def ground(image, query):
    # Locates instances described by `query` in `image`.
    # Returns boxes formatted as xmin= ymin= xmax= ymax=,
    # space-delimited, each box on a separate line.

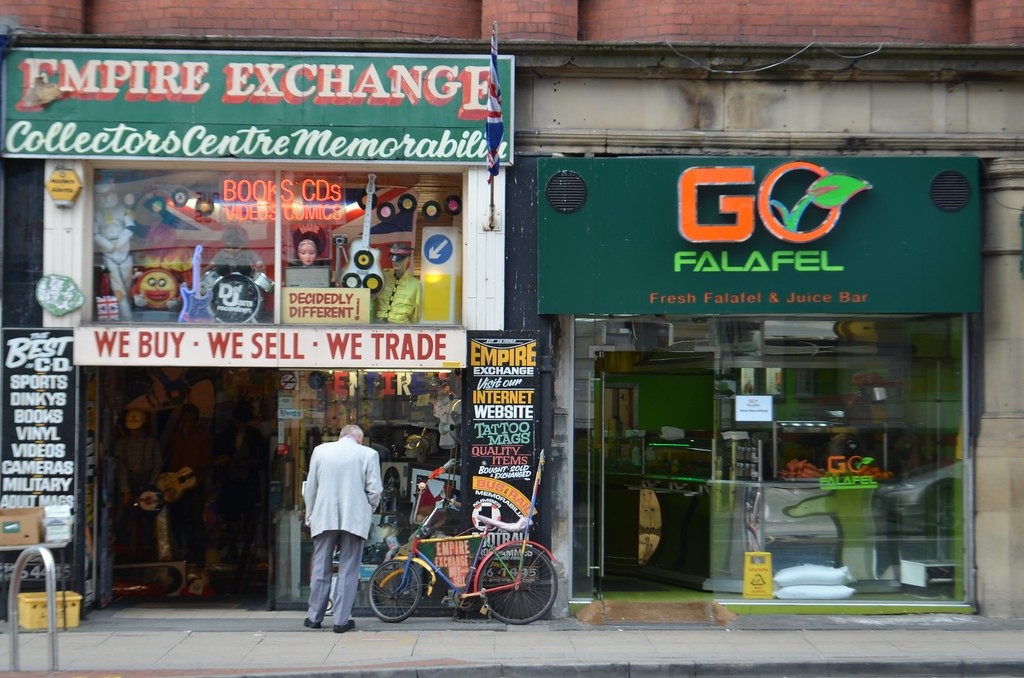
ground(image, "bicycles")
xmin=367 ymin=481 xmax=559 ymax=625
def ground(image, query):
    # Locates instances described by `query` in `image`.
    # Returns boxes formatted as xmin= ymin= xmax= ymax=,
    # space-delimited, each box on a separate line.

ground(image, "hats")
xmin=125 ymin=411 xmax=146 ymax=429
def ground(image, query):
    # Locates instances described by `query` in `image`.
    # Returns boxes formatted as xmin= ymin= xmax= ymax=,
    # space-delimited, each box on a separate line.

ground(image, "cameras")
xmin=333 ymin=235 xmax=348 ymax=245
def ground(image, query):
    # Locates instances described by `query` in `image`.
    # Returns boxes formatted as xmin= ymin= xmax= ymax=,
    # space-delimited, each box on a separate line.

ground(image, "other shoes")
xmin=221 ymin=547 xmax=253 ymax=564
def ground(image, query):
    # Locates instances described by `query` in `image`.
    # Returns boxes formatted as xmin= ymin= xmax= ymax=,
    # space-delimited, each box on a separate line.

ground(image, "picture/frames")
xmin=379 ymin=457 xmax=462 ymax=505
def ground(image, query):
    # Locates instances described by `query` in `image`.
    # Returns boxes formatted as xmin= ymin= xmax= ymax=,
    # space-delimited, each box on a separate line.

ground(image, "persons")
xmin=201 ymin=228 xmax=266 ymax=278
xmin=93 ymin=182 xmax=134 ymax=319
xmin=298 ymin=239 xmax=422 ymax=325
xmin=113 ymin=404 xmax=270 ymax=578
xmin=303 ymin=425 xmax=384 ymax=633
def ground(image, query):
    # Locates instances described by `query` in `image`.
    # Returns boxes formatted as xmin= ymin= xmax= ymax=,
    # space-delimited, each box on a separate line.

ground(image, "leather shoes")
xmin=333 ymin=620 xmax=354 ymax=633
xmin=305 ymin=618 xmax=321 ymax=629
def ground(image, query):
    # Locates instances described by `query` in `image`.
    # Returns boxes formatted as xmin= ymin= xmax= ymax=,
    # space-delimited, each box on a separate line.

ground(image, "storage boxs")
xmin=19 ymin=590 xmax=83 ymax=629
xmin=0 ymin=507 xmax=43 ymax=545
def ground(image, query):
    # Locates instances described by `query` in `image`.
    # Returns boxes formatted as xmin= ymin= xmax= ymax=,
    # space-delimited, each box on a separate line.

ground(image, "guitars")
xmin=338 ymin=173 xmax=386 ymax=296
xmin=177 ymin=244 xmax=215 ymax=323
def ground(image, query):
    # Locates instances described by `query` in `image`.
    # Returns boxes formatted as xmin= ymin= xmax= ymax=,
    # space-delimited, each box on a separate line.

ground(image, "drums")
xmin=252 ymin=273 xmax=275 ymax=295
xmin=199 ymin=270 xmax=220 ymax=293
xmin=212 ymin=272 xmax=261 ymax=324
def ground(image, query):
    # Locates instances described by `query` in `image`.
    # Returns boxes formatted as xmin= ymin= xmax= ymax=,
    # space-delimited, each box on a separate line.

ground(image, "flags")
xmin=486 ymin=21 xmax=503 ymax=185
